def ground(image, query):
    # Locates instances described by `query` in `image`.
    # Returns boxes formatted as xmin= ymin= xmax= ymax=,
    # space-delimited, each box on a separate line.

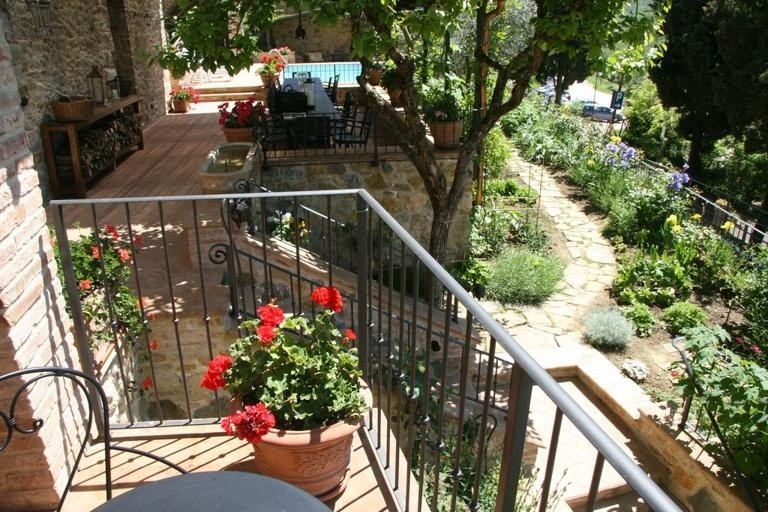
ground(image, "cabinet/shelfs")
xmin=40 ymin=94 xmax=143 ymax=200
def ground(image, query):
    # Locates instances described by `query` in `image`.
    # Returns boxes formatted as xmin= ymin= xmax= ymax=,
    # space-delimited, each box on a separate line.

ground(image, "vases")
xmin=173 ymin=100 xmax=188 ymax=113
xmin=251 ymin=378 xmax=373 ymax=504
xmin=282 ymin=55 xmax=288 ymax=62
xmin=260 ymin=75 xmax=278 ymax=87
xmin=222 ymin=127 xmax=254 ymax=143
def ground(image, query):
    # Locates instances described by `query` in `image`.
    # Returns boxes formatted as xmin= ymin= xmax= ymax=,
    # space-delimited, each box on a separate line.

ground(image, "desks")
xmin=282 ymin=61 xmax=363 ymax=85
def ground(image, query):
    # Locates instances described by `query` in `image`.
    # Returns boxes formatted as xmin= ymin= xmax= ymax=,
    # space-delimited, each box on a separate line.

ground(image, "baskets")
xmin=49 ymin=96 xmax=96 ymax=123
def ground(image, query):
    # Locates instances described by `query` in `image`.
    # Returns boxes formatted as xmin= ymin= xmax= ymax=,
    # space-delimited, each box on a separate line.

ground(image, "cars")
xmin=537 ymin=84 xmax=624 ymax=124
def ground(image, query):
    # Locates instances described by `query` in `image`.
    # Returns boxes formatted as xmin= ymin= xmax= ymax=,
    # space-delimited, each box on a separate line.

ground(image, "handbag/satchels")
xmin=273 ymin=91 xmax=308 ymax=112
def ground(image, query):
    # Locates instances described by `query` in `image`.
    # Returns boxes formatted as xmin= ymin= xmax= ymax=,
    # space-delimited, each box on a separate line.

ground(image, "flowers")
xmin=202 ymin=286 xmax=376 ymax=444
xmin=170 ymin=84 xmax=200 ymax=105
xmin=218 ymin=98 xmax=267 ymax=127
xmin=254 ymin=54 xmax=286 ymax=75
xmin=279 ymin=46 xmax=289 ymax=55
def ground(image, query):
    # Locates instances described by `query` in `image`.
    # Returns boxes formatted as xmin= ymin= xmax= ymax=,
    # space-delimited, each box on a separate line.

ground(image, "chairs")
xmin=0 ymin=366 xmax=334 ymax=512
xmin=259 ymin=72 xmax=376 ymax=152
xmin=305 ymin=51 xmax=345 ymax=62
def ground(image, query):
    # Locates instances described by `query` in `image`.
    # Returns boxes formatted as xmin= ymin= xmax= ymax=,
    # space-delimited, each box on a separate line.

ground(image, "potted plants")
xmin=367 ymin=62 xmax=465 ymax=149
